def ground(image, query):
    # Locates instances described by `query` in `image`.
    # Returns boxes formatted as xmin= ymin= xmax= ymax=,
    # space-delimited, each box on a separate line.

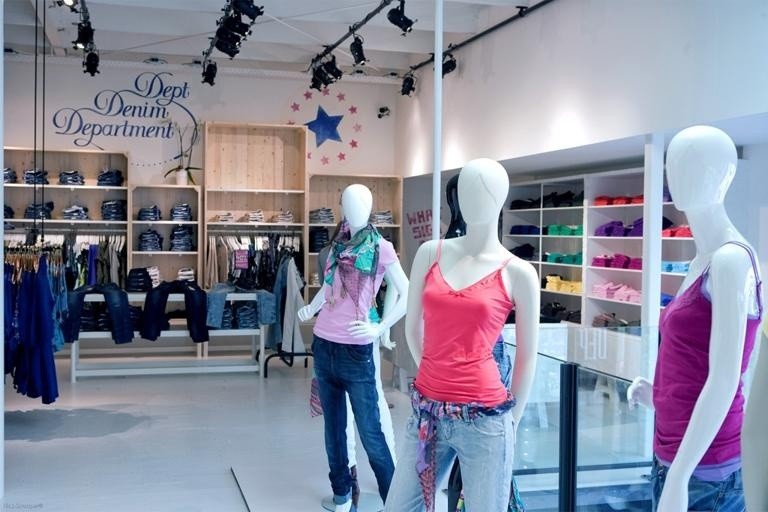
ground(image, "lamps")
xmin=56 ymin=0 xmax=100 ymax=77
xmin=309 ymin=0 xmax=417 ymax=96
xmin=442 ymin=51 xmax=456 ymax=79
xmin=201 ymin=0 xmax=264 ymax=86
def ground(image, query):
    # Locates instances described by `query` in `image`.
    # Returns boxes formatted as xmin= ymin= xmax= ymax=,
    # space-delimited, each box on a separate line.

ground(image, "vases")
xmin=173 ymin=144 xmax=192 ymax=185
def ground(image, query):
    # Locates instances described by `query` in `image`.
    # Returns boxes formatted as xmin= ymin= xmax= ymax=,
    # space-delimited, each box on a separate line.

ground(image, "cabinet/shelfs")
xmin=307 ymin=171 xmax=404 ymax=305
xmin=129 ymin=185 xmax=202 ymax=288
xmin=70 ymin=291 xmax=270 ymax=384
xmin=203 ymin=120 xmax=309 ymax=303
xmin=2 ymin=145 xmax=131 ymax=279
xmin=499 ymin=165 xmax=696 ymax=382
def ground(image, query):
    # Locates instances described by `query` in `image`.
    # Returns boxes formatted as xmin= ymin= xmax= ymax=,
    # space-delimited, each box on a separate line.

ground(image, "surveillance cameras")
xmin=378 ymin=106 xmax=390 ymax=118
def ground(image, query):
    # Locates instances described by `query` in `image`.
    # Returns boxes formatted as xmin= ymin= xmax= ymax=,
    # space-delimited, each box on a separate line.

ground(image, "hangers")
xmin=3 ymin=228 xmax=126 ymax=285
xmin=208 ymin=229 xmax=301 ymax=253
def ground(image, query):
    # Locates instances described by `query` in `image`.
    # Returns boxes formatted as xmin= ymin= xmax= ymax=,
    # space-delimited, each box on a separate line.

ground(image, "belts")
xmin=410 ymin=380 xmax=517 ymax=512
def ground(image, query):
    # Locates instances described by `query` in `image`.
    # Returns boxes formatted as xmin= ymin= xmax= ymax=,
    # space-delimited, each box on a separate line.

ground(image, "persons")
xmin=625 ymin=124 xmax=763 ymax=511
xmin=380 ymin=155 xmax=539 ymax=511
xmin=293 ymin=181 xmax=410 ymax=511
